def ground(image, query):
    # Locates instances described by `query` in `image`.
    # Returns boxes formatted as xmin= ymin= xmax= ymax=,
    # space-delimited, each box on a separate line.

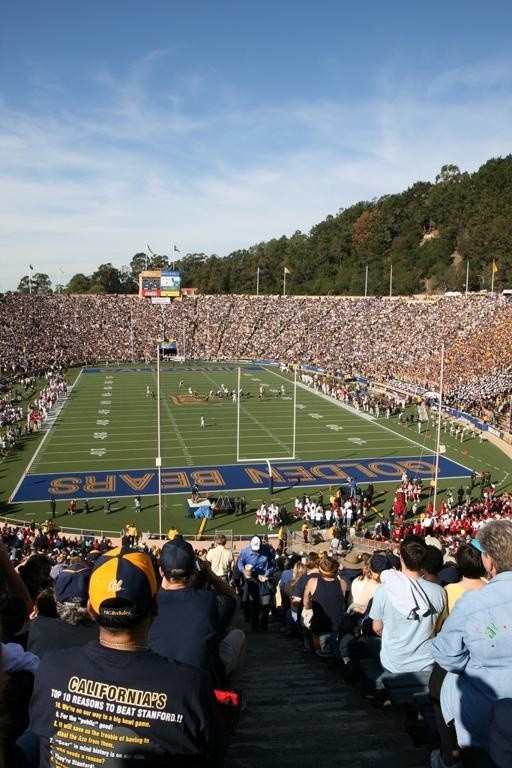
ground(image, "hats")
xmin=88 ymin=545 xmax=158 ymax=616
xmin=339 ymin=551 xmax=364 ymax=570
xmin=159 ymin=538 xmax=195 ymax=578
xmin=471 ymin=538 xmax=486 ymax=553
xmin=54 ymin=561 xmax=92 ymax=607
xmin=250 ymin=534 xmax=262 ymax=551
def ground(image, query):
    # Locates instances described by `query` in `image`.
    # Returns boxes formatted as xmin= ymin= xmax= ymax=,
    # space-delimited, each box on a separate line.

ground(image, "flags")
xmin=492 ymin=258 xmax=500 ymax=273
xmin=28 ymin=263 xmax=34 ymax=273
xmin=57 ymin=263 xmax=65 ymax=275
xmin=284 ymin=267 xmax=292 ymax=275
xmin=173 ymin=241 xmax=181 ymax=255
xmin=145 ymin=242 xmax=152 ymax=255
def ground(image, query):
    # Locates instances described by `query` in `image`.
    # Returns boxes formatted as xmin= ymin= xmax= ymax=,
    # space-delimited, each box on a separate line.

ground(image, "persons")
xmin=28 ymin=590 xmax=60 ymax=622
xmin=421 ymin=545 xmax=444 ymax=587
xmin=18 ymin=564 xmax=91 ymax=659
xmin=368 ymin=534 xmax=450 ymax=694
xmin=431 ymin=520 xmax=512 ymax=767
xmin=147 ymin=540 xmax=246 ymax=693
xmin=0 ymin=517 xmax=207 ymax=569
xmin=444 ymin=542 xmax=488 ymax=616
xmin=305 ymin=552 xmax=321 ymax=575
xmin=273 ymin=557 xmax=291 ymax=594
xmin=206 ymin=535 xmax=235 ymax=586
xmin=279 ymin=555 xmax=302 ymax=603
xmin=302 ymin=556 xmax=347 ymax=658
xmin=340 ymin=553 xmax=365 ymax=575
xmin=279 ymin=561 xmax=307 ymax=640
xmin=28 ymin=545 xmax=230 ymax=768
xmin=44 ymin=484 xmax=248 ymax=518
xmin=337 ymin=554 xmax=392 ymax=641
xmin=0 ymin=536 xmax=39 ymax=621
xmin=254 ymin=469 xmax=512 ymax=554
xmin=238 ymin=536 xmax=279 ymax=631
xmin=0 ymin=289 xmax=512 ymax=458
